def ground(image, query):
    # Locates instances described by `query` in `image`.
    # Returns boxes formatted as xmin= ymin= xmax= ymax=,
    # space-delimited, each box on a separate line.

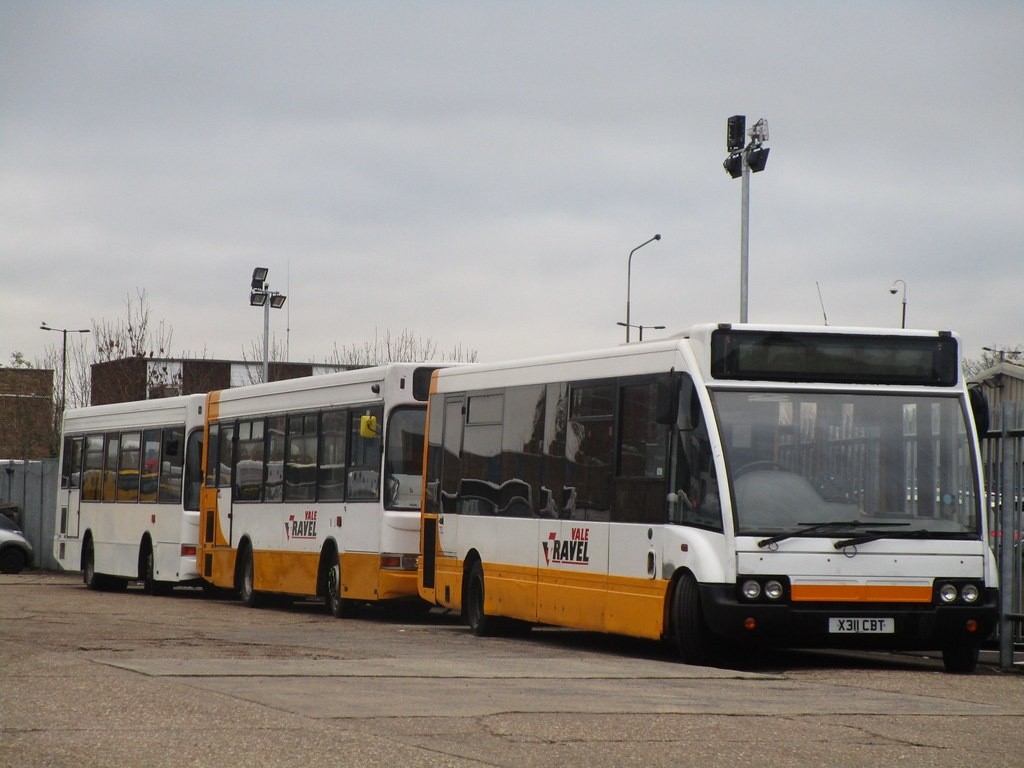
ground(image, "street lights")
xmin=249 ymin=266 xmax=287 ymax=383
xmin=627 ymin=233 xmax=662 ymax=343
xmin=890 ymin=278 xmax=907 ymax=329
xmin=40 ymin=326 xmax=91 ymax=415
xmin=616 ymin=322 xmax=665 ymax=342
xmin=981 ymin=347 xmax=1021 ymax=363
xmin=6 ymin=468 xmax=15 ymax=504
xmin=723 ymin=116 xmax=770 ymax=321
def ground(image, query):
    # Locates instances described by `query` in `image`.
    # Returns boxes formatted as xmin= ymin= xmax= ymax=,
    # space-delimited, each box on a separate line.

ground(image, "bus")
xmin=415 ymin=321 xmax=1000 ymax=674
xmin=196 ymin=363 xmax=474 ymax=617
xmin=53 ymin=394 xmax=210 ymax=595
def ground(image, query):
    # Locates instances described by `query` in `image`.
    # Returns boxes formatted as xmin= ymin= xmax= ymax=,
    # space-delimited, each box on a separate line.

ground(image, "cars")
xmin=0 ymin=513 xmax=35 ymax=573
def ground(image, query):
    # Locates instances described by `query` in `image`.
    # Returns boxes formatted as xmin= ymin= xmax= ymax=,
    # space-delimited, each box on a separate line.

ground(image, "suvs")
xmin=849 ymin=484 xmax=1024 ymax=548
